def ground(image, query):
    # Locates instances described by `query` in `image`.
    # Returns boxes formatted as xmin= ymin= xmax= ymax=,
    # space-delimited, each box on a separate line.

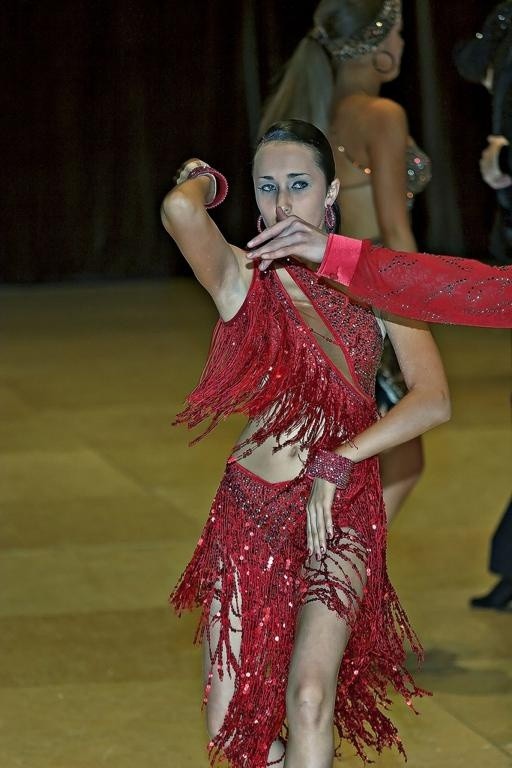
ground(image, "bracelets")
xmin=197 ymin=172 xmax=218 ymax=206
xmin=304 ymin=447 xmax=355 ymax=493
xmin=183 ymin=165 xmax=230 ymax=211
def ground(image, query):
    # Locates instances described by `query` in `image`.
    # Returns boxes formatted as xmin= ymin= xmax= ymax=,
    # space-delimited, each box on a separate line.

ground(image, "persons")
xmin=254 ymin=0 xmax=443 ymax=535
xmin=468 ymin=500 xmax=512 ymax=613
xmin=478 ymin=133 xmax=512 ymax=192
xmin=242 ymin=205 xmax=512 ymax=334
xmin=155 ymin=116 xmax=458 ymax=768
